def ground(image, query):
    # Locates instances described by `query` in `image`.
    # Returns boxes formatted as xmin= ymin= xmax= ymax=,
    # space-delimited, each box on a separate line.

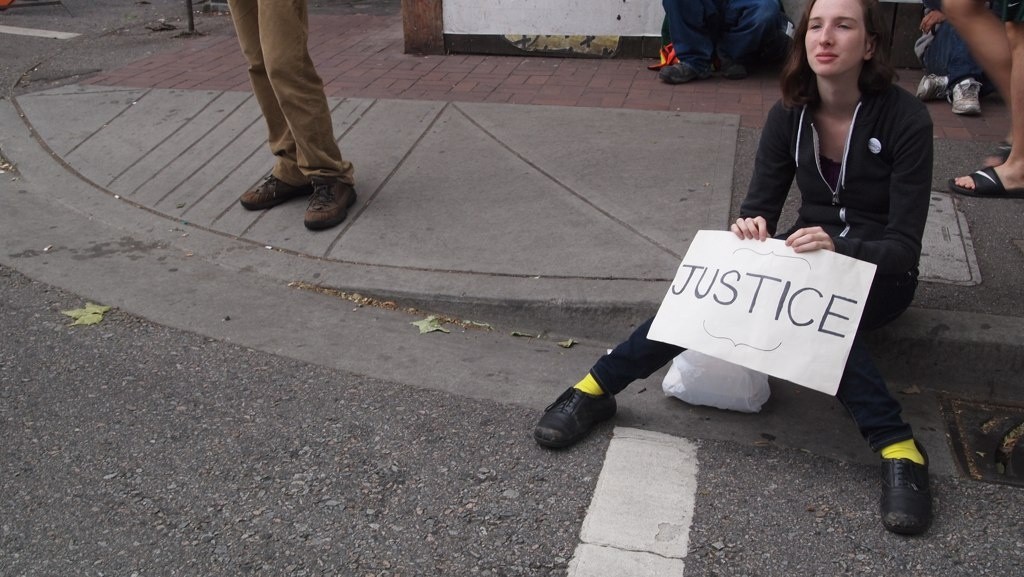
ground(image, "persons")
xmin=225 ymin=0 xmax=356 ymax=230
xmin=534 ymin=0 xmax=933 ymax=536
xmin=941 ymin=0 xmax=1024 ymax=199
xmin=659 ymin=0 xmax=808 ymax=84
xmin=915 ymin=0 xmax=1003 ymax=114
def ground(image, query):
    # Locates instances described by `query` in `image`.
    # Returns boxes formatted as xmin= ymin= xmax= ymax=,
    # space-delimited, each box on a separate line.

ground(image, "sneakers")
xmin=304 ymin=180 xmax=358 ymax=230
xmin=952 ymin=77 xmax=983 ymax=116
xmin=240 ymin=174 xmax=314 ymax=211
xmin=659 ymin=61 xmax=713 ymax=85
xmin=915 ymin=73 xmax=952 ymax=104
xmin=720 ymin=64 xmax=748 ymax=80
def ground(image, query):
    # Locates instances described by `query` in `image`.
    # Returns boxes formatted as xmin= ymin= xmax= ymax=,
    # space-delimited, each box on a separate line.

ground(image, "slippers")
xmin=986 ymin=138 xmax=1013 ymax=165
xmin=948 ymin=164 xmax=1024 ymax=199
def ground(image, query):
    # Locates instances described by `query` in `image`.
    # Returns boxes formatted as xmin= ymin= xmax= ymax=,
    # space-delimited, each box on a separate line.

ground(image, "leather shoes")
xmin=534 ymin=386 xmax=616 ymax=449
xmin=880 ymin=440 xmax=934 ymax=535
